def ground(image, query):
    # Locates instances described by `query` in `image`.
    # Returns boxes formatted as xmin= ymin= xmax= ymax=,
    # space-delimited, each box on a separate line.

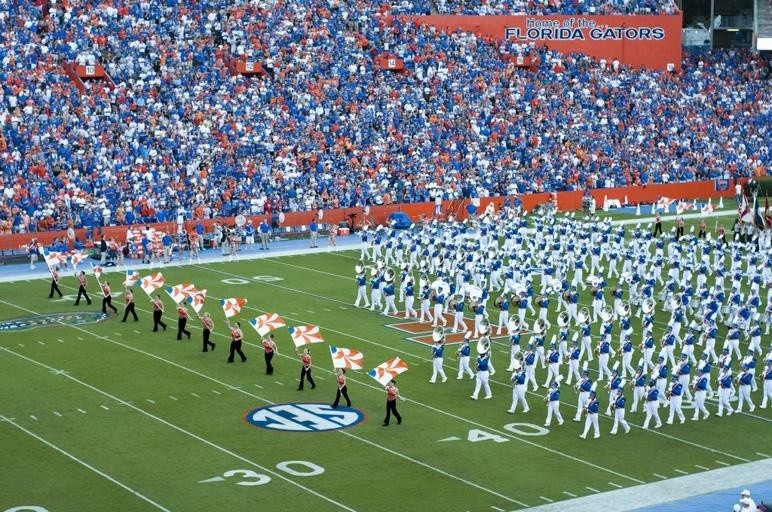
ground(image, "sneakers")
xmin=354 ymin=210 xmax=772 ymax=440
xmin=46 ymin=295 xmax=351 ymax=409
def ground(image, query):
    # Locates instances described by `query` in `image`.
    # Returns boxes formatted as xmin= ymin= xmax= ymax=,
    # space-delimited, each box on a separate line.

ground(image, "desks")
xmin=605 ymin=199 xmax=622 ymax=209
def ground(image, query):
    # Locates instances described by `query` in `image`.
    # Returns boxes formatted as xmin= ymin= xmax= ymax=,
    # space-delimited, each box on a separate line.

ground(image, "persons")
xmin=47 ymin=264 xmax=403 ymax=428
xmin=354 ymin=240 xmax=771 ymax=440
xmin=0 ymin=0 xmax=772 ymax=271
xmin=733 ymin=487 xmax=772 ymax=512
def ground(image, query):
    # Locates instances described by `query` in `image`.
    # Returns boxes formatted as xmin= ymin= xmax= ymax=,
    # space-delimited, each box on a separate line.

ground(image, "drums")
xmin=432 ymin=280 xmax=450 ymax=297
xmin=691 ymin=295 xmax=768 ymax=330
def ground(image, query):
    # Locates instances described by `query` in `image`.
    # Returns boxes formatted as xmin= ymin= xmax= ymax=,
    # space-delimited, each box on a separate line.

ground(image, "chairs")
xmin=0 ymin=223 xmax=331 ymax=264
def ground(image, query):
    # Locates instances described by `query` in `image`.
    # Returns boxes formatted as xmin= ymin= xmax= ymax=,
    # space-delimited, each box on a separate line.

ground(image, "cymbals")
xmin=428 ymin=287 xmax=618 ymax=313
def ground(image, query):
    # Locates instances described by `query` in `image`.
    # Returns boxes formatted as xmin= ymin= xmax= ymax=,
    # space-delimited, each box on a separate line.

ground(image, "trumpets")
xmin=543 ymin=326 xmax=772 ymax=416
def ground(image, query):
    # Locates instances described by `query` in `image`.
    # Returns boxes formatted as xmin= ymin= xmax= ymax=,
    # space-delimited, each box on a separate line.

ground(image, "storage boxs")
xmin=338 ymin=220 xmax=349 ymax=227
xmin=338 ymin=226 xmax=350 ymax=236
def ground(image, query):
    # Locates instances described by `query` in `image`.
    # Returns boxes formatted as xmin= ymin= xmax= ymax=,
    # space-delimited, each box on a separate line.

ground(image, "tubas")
xmin=556 ymin=292 xmax=683 ymax=344
xmin=508 ymin=292 xmax=550 ymax=387
xmin=433 ymin=319 xmax=493 ymax=372
xmin=354 ymin=256 xmax=431 ymax=296
